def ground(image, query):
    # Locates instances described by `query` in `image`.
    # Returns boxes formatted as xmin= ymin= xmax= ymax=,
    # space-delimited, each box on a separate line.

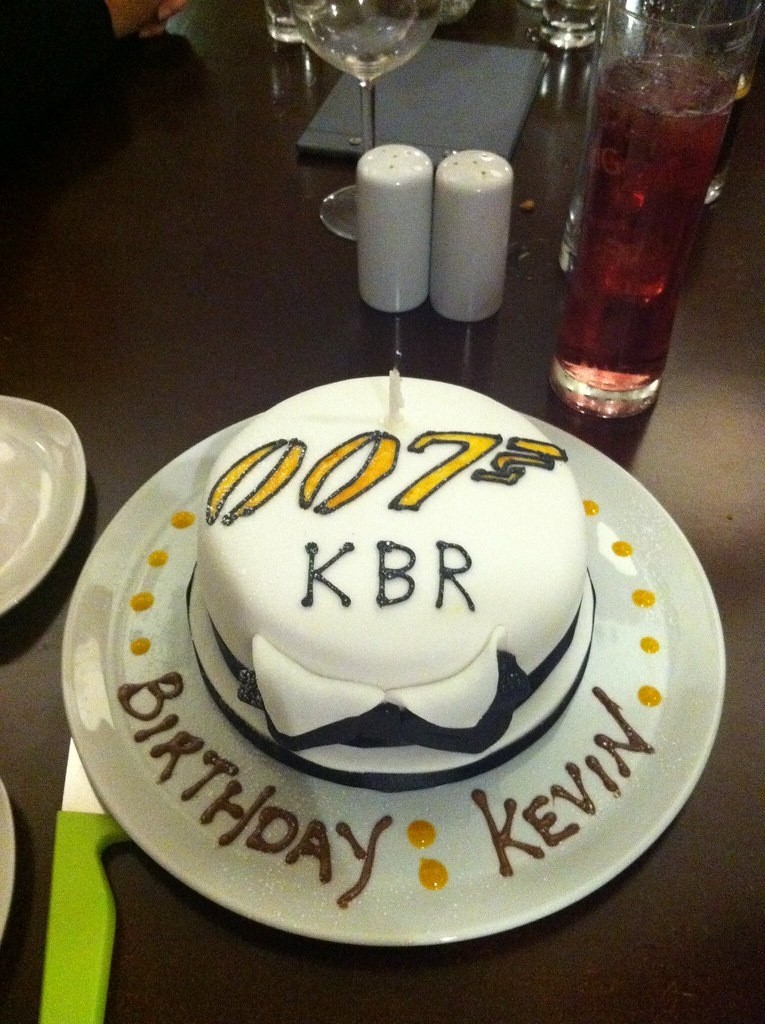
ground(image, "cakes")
xmin=184 ymin=376 xmax=599 ymax=794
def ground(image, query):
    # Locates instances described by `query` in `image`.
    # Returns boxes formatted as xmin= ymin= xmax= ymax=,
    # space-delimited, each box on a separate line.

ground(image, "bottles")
xmin=430 ymin=149 xmax=514 ymax=321
xmin=356 ymin=144 xmax=434 ymax=313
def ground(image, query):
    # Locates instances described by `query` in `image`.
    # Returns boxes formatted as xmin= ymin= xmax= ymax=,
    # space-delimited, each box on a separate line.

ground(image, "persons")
xmin=0 ymin=0 xmax=184 ymax=183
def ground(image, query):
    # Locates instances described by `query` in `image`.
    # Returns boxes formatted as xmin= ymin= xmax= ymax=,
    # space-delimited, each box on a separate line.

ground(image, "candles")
xmin=390 ymin=351 xmax=404 ymax=424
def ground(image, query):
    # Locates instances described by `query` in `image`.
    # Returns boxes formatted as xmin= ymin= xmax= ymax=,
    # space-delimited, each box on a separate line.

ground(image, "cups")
xmin=264 ymin=0 xmax=303 ymax=44
xmin=538 ymin=0 xmax=599 ymax=51
xmin=546 ymin=0 xmax=762 ymax=420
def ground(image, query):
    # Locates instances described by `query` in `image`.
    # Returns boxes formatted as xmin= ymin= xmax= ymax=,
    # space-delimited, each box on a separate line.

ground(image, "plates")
xmin=60 ymin=420 xmax=725 ymax=943
xmin=0 ymin=393 xmax=87 ymax=613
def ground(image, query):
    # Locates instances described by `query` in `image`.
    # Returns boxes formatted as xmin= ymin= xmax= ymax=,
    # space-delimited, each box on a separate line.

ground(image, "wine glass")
xmin=289 ymin=1 xmax=440 ymax=241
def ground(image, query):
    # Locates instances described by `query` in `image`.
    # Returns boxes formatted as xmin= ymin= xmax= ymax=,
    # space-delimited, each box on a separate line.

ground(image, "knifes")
xmin=39 ymin=739 xmax=131 ymax=1022
